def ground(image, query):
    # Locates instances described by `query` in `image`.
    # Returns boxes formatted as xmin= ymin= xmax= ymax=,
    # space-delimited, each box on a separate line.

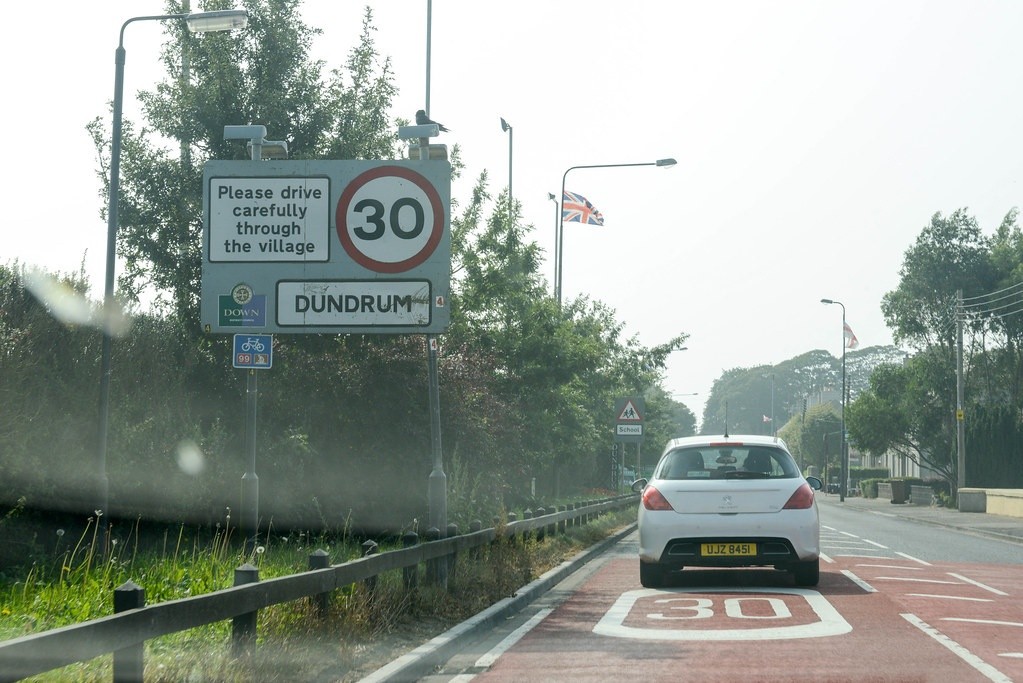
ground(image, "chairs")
xmin=745 ymin=449 xmax=774 ymax=476
xmin=671 ymin=451 xmax=704 ymax=477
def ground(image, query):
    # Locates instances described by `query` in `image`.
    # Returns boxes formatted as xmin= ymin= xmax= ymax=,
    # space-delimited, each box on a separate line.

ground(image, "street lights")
xmin=92 ymin=5 xmax=252 ymax=584
xmin=820 ymin=298 xmax=848 ymax=502
xmin=557 ymin=158 xmax=680 ymax=308
xmin=500 ymin=116 xmax=514 ymax=224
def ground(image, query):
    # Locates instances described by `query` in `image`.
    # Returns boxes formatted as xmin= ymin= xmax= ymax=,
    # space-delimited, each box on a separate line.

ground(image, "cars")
xmin=631 ymin=433 xmax=821 ymax=588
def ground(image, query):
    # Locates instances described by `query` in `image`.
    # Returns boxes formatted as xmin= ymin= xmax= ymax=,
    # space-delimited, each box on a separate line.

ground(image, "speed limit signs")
xmin=335 ymin=166 xmax=444 ymax=273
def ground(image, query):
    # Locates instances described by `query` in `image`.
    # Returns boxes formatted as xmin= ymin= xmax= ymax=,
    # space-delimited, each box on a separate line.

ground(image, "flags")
xmin=844 ymin=322 xmax=860 ymax=349
xmin=562 ymin=190 xmax=604 ymax=226
